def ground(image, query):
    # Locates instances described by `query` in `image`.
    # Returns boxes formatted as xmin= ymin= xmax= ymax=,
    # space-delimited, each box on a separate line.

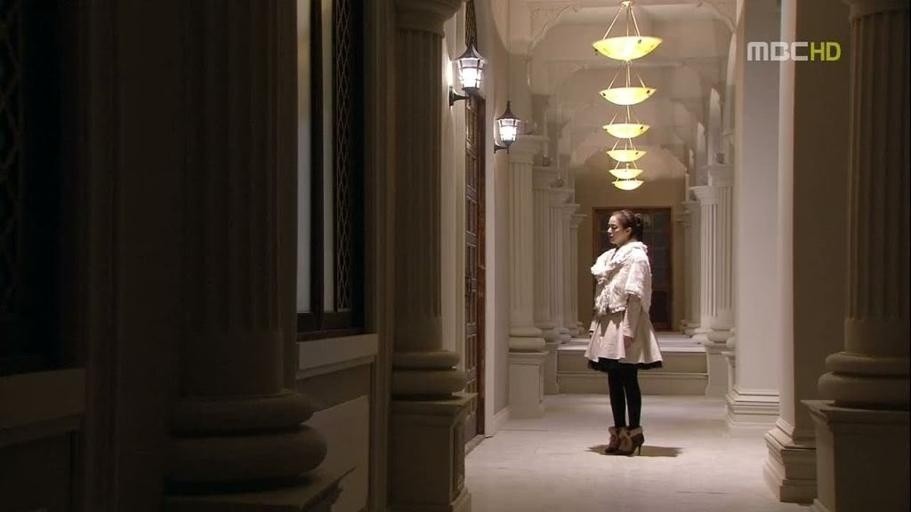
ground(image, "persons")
xmin=584 ymin=210 xmax=664 ymax=458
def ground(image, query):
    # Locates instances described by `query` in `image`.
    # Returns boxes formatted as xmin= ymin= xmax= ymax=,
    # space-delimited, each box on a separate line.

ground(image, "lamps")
xmin=447 ymin=2 xmax=666 ymax=191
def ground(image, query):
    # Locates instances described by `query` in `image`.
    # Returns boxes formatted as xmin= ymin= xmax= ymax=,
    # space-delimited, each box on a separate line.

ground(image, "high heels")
xmin=605 ymin=426 xmax=644 ymax=456
